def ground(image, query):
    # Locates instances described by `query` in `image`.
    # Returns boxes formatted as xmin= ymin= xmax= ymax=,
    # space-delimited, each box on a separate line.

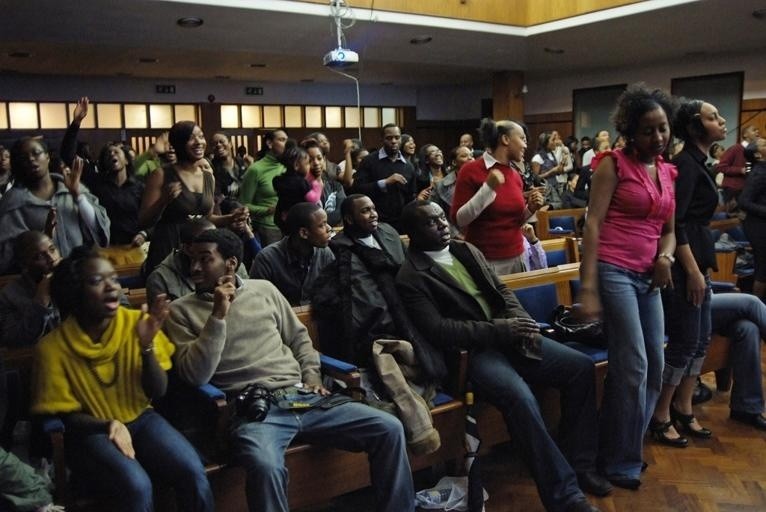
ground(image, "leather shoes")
xmin=730 ymin=408 xmax=766 ymax=430
xmin=567 ymin=460 xmax=647 ymax=512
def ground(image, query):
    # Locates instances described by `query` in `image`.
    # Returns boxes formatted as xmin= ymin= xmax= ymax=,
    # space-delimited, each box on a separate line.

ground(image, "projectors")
xmin=323 ymin=49 xmax=360 ymax=66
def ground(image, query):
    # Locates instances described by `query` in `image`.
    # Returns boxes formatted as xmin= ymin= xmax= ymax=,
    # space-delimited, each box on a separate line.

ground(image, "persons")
xmin=1 ymin=81 xmax=766 ymax=511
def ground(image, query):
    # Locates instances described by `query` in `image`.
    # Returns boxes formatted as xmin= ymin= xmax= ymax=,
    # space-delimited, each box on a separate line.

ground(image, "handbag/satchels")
xmin=553 ymin=303 xmax=609 ymax=351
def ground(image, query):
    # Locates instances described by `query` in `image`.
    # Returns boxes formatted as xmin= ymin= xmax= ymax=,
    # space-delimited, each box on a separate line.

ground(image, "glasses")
xmin=424 ymin=150 xmax=442 ymax=160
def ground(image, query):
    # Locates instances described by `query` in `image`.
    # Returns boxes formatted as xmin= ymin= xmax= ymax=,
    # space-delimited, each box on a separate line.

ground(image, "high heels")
xmin=669 ymin=403 xmax=712 ymax=438
xmin=649 ymin=414 xmax=689 ymax=447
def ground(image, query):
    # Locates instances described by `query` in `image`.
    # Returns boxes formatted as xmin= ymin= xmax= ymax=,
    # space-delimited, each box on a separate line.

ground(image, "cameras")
xmin=234 ymin=383 xmax=274 ymax=422
